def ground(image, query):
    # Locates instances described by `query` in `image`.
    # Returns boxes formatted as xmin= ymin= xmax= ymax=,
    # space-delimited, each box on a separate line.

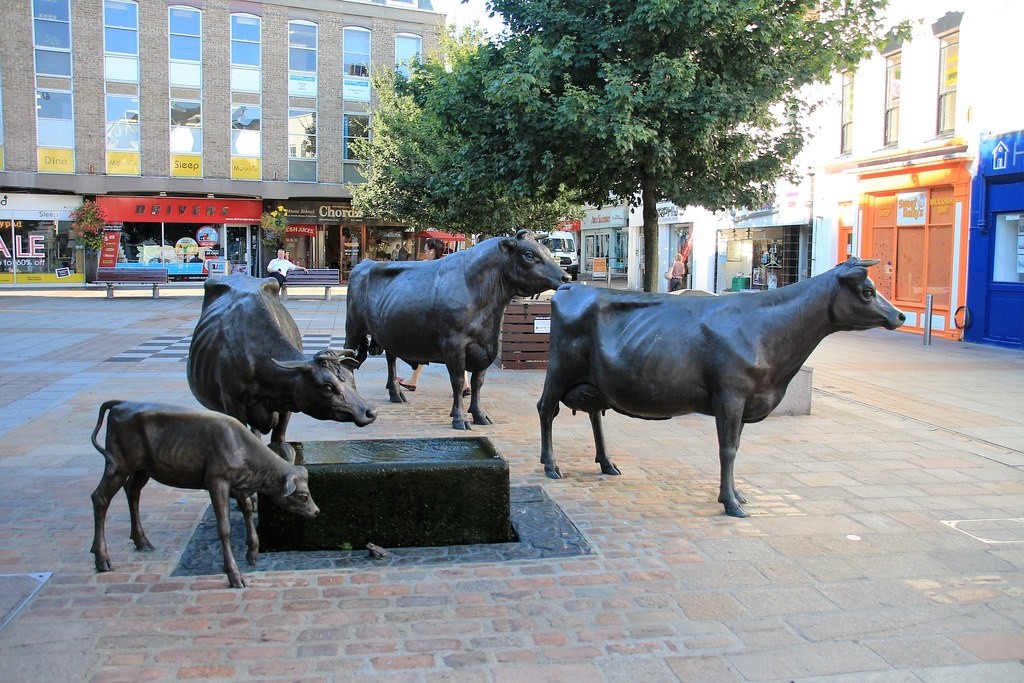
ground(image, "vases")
xmin=85 ymin=245 xmax=96 ymax=283
xmin=263 ymin=246 xmax=276 ymax=278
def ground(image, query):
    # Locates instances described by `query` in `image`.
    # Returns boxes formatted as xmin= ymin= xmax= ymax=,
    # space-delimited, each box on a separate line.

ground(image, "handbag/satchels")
xmin=664 ymin=261 xmax=675 ymax=280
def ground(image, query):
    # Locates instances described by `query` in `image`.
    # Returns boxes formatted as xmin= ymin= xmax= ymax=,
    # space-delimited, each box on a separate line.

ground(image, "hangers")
xmin=765 ymin=258 xmax=782 ymax=269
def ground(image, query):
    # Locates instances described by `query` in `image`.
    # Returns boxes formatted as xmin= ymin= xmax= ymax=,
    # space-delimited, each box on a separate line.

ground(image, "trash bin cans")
xmin=208 ymin=260 xmax=229 ymax=280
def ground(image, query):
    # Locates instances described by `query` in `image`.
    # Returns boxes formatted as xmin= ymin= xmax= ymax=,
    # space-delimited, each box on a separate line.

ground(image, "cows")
xmin=90 ymin=229 xmax=907 ymax=590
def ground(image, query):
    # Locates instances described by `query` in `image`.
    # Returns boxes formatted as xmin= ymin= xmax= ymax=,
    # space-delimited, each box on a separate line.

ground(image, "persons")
xmin=668 ymin=253 xmax=684 ymax=292
xmin=554 ymin=240 xmax=565 ymax=251
xmin=267 ymin=249 xmax=308 ymax=290
xmin=444 ymin=243 xmax=453 ymax=254
xmin=577 ymin=248 xmax=581 ymax=274
xmin=396 ymin=238 xmax=472 ymax=397
xmin=232 ymin=260 xmax=244 ymax=273
xmin=391 ymin=243 xmax=411 ymax=261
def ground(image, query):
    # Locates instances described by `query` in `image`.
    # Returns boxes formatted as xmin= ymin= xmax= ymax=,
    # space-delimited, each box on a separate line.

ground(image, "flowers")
xmin=69 ymin=199 xmax=109 ymax=253
xmin=259 ymin=205 xmax=288 ymax=249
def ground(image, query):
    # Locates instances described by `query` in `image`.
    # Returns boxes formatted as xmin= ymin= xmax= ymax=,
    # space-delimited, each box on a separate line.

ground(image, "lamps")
xmin=159 ymin=191 xmax=167 ymax=197
xmin=207 ymin=193 xmax=214 ymax=199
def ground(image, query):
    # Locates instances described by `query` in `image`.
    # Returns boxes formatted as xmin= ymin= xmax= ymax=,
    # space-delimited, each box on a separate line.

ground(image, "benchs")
xmin=91 ymin=267 xmax=168 ymax=299
xmin=279 ymin=269 xmax=340 ymax=302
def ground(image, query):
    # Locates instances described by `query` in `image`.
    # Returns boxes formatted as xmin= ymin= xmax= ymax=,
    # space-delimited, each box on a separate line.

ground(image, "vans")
xmin=535 ymin=230 xmax=578 ymax=281
